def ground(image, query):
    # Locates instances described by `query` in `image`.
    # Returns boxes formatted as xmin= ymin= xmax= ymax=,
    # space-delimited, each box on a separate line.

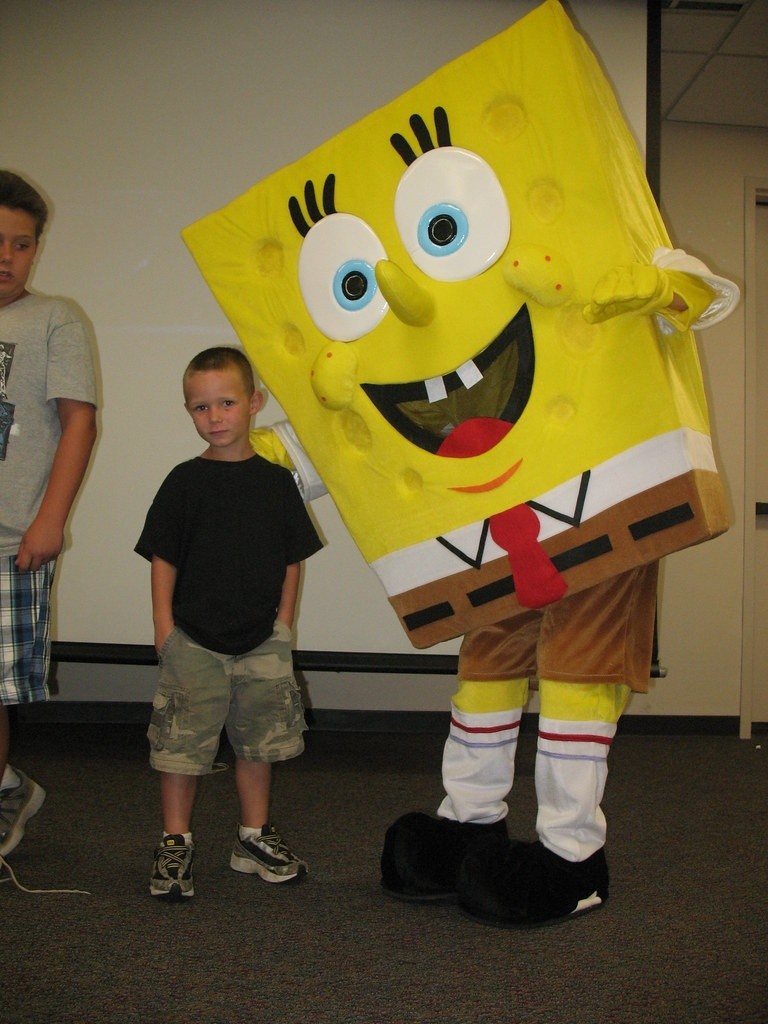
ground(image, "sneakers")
xmin=230 ymin=824 xmax=308 ymax=883
xmin=150 ymin=833 xmax=195 ymax=900
xmin=0 ymin=765 xmax=91 ymax=895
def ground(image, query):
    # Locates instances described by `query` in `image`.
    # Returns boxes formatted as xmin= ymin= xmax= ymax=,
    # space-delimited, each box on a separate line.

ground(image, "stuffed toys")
xmin=181 ymin=0 xmax=742 ymax=929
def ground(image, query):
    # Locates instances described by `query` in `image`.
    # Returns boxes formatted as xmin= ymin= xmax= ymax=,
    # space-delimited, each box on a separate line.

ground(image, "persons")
xmin=0 ymin=168 xmax=98 ymax=855
xmin=133 ymin=345 xmax=323 ymax=901
